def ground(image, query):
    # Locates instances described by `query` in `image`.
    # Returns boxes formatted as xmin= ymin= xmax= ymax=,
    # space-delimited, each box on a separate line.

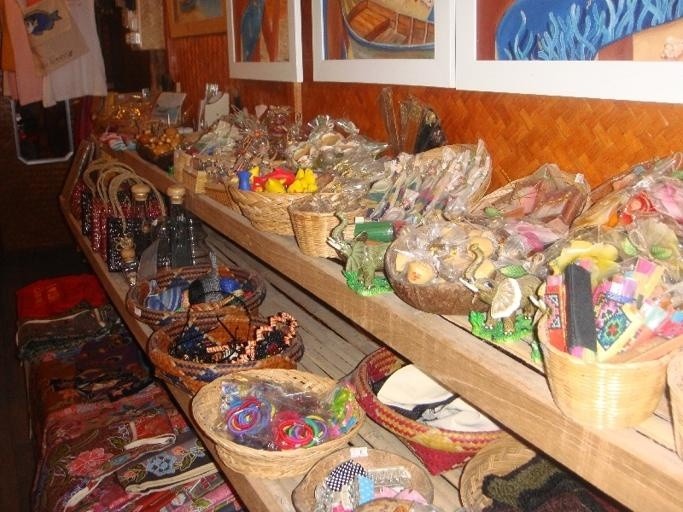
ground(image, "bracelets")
xmin=219 ymin=381 xmax=358 ymax=451
xmin=166 ymin=309 xmax=298 ymax=366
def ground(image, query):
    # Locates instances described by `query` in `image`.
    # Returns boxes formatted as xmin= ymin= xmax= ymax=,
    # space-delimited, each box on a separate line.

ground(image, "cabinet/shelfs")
xmin=0 ymin=96 xmax=683 ymax=512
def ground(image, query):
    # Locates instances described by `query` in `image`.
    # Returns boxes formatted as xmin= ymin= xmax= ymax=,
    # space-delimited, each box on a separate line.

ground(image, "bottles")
xmin=121 ymin=247 xmax=140 ymax=289
xmin=125 ymin=184 xmax=157 ymax=262
xmin=150 ymin=186 xmax=212 ymax=271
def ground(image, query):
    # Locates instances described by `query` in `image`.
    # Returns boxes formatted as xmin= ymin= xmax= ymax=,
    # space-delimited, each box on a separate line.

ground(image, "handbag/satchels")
xmin=71 ymin=156 xmax=167 ymax=274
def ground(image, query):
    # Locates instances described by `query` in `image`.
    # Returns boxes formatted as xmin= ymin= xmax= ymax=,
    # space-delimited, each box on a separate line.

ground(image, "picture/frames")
xmin=164 ymin=2 xmax=227 ymax=40
xmin=226 ymin=1 xmax=304 ymax=82
xmin=312 ymin=0 xmax=456 ymax=89
xmin=455 ymin=3 xmax=683 ymax=105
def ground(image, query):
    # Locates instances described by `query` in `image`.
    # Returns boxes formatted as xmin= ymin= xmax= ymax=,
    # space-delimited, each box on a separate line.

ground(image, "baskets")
xmin=294 ymin=444 xmax=433 ymax=512
xmin=409 ymin=143 xmax=493 ymax=211
xmin=461 ymin=440 xmax=541 ymax=512
xmin=536 ymin=308 xmax=680 ymax=431
xmin=384 ymin=222 xmax=489 ymax=315
xmin=235 ymin=160 xmax=368 ymax=237
xmin=147 ymin=312 xmax=304 ymax=396
xmin=350 ymin=345 xmax=510 ymax=477
xmin=124 ymin=263 xmax=266 ymax=334
xmin=469 ymin=163 xmax=592 ymax=236
xmin=287 ymin=195 xmax=376 ymax=260
xmin=94 ymin=109 xmax=275 ymax=191
xmin=187 ymin=368 xmax=367 ymax=480
xmin=666 ymin=351 xmax=683 ymax=458
xmin=233 ymin=154 xmax=591 ymax=316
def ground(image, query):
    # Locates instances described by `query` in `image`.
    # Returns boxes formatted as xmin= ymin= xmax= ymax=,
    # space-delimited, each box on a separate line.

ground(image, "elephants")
xmin=460 ymin=242 xmax=544 ymax=337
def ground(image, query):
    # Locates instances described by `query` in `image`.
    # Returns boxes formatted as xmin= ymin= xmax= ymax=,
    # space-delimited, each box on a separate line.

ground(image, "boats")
xmin=338 ymin=1 xmax=434 ymax=52
